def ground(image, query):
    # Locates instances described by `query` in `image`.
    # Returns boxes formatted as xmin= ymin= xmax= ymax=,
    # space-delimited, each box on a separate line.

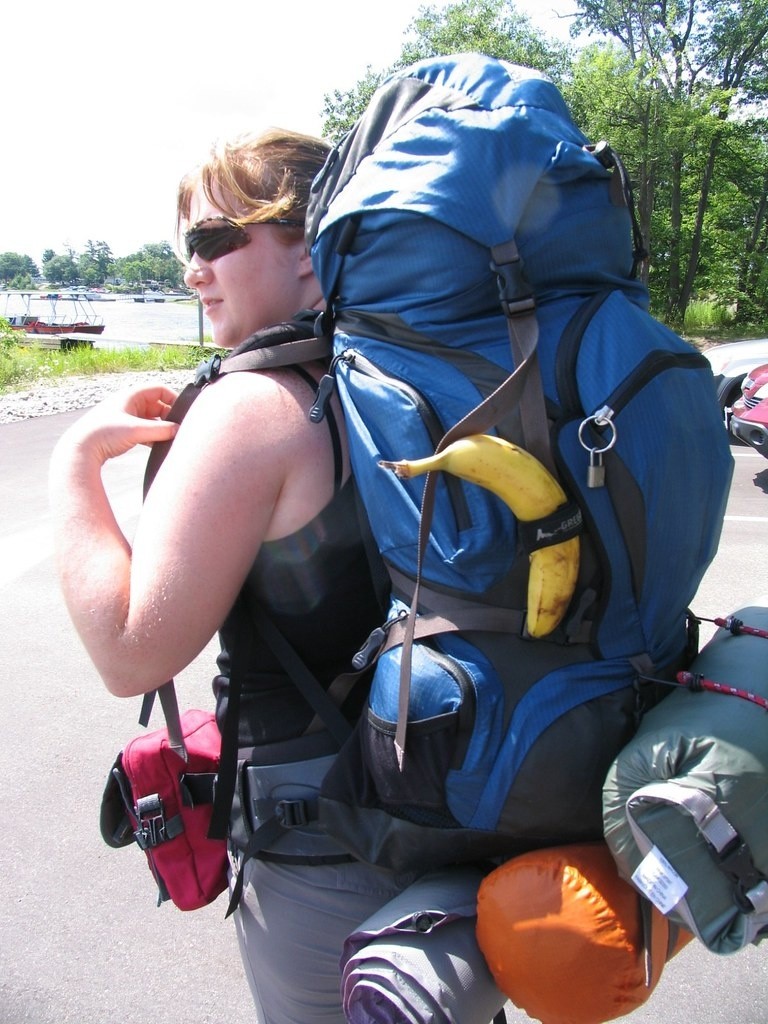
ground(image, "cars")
xmin=702 ymin=338 xmax=768 ymax=432
xmin=725 ymin=363 xmax=768 ymax=459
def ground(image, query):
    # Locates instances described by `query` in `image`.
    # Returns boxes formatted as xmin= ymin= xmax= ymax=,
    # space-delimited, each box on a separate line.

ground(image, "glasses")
xmin=185 ymin=218 xmax=307 ymax=266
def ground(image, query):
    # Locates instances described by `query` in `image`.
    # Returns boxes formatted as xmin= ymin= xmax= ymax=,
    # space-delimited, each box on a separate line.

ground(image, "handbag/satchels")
xmin=99 ymin=711 xmax=232 ymax=911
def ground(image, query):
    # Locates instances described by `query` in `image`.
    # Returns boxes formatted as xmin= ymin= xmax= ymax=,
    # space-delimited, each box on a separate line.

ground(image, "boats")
xmin=1 ymin=287 xmax=107 ymax=337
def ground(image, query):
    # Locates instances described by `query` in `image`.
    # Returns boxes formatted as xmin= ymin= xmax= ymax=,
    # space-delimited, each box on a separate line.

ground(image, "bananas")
xmin=392 ymin=433 xmax=580 ymax=638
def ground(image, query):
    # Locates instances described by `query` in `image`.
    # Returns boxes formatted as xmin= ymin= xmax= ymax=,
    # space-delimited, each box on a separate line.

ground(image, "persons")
xmin=52 ymin=128 xmax=513 ymax=1024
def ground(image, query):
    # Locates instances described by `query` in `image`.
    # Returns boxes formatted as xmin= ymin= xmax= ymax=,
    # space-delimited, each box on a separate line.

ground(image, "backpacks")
xmin=195 ymin=51 xmax=732 ymax=874
